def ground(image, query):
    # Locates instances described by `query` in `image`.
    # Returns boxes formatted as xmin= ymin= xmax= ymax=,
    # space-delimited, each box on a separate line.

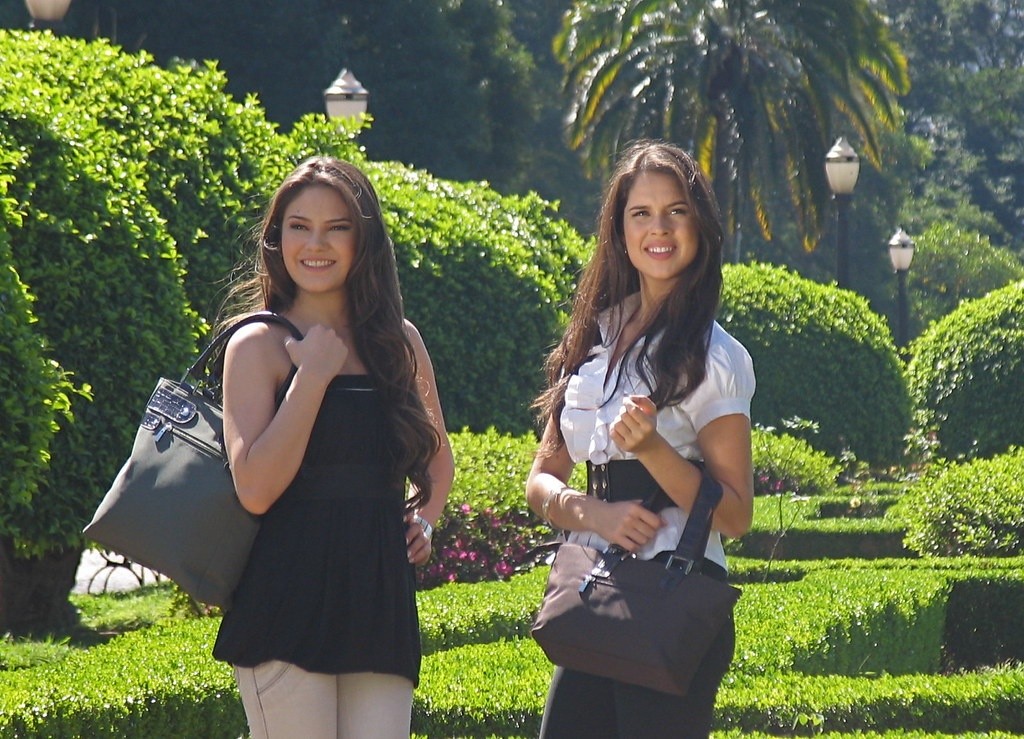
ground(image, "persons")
xmin=212 ymin=155 xmax=454 ymax=738
xmin=525 ymin=143 xmax=757 ymax=739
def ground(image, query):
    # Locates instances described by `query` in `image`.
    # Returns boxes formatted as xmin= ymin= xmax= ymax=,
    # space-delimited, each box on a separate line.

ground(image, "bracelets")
xmin=414 ymin=515 xmax=433 ymax=537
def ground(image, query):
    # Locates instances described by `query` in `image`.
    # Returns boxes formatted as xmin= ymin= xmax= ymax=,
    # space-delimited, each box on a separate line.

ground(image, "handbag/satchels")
xmin=81 ymin=311 xmax=306 ymax=612
xmin=530 ymin=457 xmax=742 ymax=696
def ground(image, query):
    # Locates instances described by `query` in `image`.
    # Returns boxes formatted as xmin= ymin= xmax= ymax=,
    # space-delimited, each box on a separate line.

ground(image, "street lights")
xmin=888 ymin=223 xmax=916 ymax=363
xmin=824 ymin=128 xmax=862 ymax=288
